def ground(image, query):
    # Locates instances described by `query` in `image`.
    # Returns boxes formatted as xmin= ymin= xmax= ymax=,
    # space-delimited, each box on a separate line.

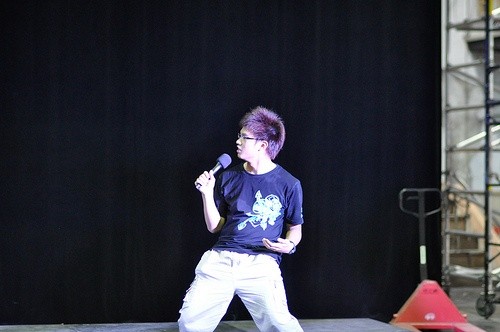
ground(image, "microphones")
xmin=198 ymin=153 xmax=232 ymax=189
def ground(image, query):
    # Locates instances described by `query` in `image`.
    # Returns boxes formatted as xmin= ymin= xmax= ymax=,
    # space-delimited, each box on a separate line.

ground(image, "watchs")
xmin=289 ymin=241 xmax=296 ymax=254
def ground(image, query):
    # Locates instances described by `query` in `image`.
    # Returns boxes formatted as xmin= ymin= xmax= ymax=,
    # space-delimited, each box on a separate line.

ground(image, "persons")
xmin=178 ymin=107 xmax=304 ymax=332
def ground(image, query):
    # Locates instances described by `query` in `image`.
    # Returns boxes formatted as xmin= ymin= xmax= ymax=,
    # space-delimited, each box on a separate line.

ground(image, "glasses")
xmin=237 ymin=132 xmax=265 ymax=141
